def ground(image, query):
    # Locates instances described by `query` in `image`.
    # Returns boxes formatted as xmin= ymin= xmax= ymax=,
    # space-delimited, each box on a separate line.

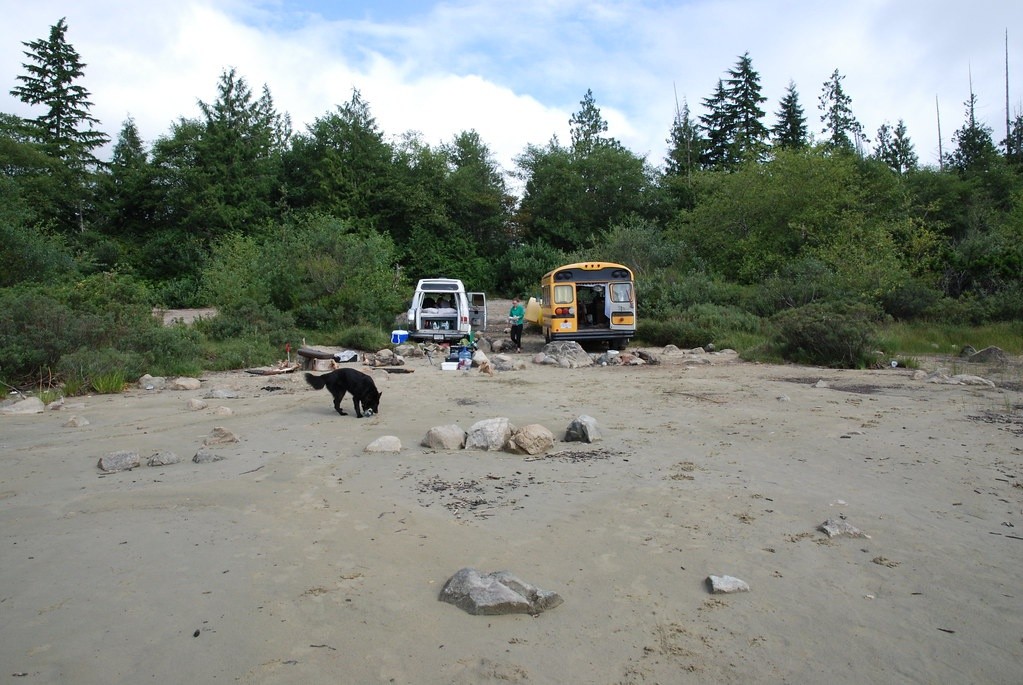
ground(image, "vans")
xmin=407 ymin=278 xmax=487 ymax=342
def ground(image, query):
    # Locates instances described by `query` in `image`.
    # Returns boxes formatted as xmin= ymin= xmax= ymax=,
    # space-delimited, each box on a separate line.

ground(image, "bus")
xmin=536 ymin=261 xmax=637 ymax=352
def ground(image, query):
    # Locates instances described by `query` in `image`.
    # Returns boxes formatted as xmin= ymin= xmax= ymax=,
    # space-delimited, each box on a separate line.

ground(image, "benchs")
xmin=295 ymin=348 xmax=337 ymax=371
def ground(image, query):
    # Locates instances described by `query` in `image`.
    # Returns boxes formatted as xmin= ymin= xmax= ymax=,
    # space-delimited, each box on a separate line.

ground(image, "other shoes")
xmin=518 ymin=347 xmax=521 ymax=353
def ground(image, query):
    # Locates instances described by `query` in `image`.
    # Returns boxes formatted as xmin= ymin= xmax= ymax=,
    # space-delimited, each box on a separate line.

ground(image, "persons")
xmin=508 ymin=298 xmax=524 ymax=353
xmin=424 ymin=295 xmax=455 ymax=329
xmin=590 ymin=288 xmax=601 ymax=325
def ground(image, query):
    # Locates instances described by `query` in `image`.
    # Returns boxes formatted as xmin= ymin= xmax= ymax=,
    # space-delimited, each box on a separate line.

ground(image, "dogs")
xmin=304 ymin=367 xmax=382 ymax=418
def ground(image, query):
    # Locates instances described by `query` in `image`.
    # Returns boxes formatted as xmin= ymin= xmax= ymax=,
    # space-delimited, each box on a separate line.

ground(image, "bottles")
xmin=459 ymin=346 xmax=472 ymax=361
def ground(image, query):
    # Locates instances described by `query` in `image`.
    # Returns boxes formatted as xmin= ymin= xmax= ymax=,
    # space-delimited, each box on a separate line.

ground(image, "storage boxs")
xmin=441 ymin=362 xmax=460 ymax=370
xmin=391 ymin=330 xmax=409 ymax=345
